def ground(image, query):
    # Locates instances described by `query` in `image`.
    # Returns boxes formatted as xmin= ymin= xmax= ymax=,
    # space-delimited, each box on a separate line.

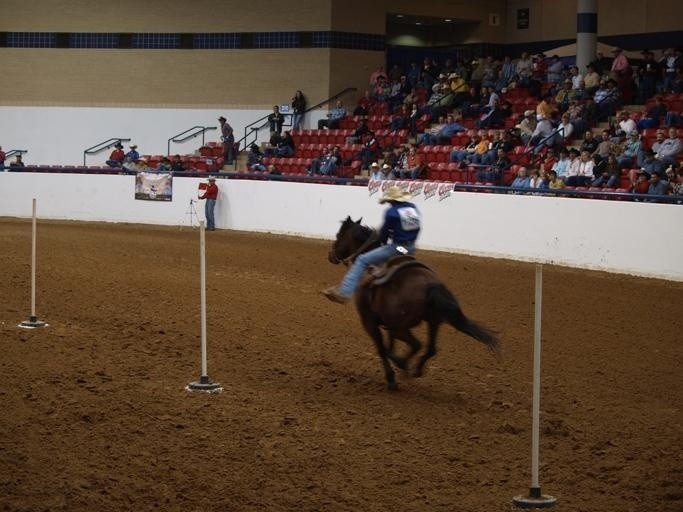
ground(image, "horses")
xmin=325 ymin=214 xmax=503 ymax=395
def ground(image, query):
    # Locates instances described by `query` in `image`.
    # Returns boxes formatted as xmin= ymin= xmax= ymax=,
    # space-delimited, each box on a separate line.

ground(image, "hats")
xmin=636 ymin=147 xmax=657 ymax=180
xmin=378 ymin=187 xmax=411 ymax=204
xmin=432 ymin=73 xmax=461 ymax=91
xmin=369 ymin=162 xmax=391 ymax=170
xmin=114 ymin=143 xmax=138 ymax=149
xmin=524 ymin=109 xmax=543 ymax=121
xmin=206 ymin=175 xmax=216 ymax=181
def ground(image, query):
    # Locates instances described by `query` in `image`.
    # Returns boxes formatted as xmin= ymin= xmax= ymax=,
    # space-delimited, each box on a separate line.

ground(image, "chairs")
xmin=227 ymin=81 xmax=681 ymax=200
xmin=26 ymin=140 xmax=227 ymax=178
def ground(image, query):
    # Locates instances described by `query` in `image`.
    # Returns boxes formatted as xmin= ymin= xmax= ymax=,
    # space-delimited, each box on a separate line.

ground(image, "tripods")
xmin=179 ymin=199 xmax=200 ymax=231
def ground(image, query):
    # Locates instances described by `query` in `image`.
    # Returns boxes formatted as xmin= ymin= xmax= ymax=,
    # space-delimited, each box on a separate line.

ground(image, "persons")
xmin=106 ymin=144 xmax=184 ymax=175
xmin=217 ymin=117 xmax=234 ymax=165
xmin=322 ymin=187 xmax=421 ymax=304
xmin=0 ymin=147 xmax=24 ymax=172
xmin=198 ymin=175 xmax=218 ymax=231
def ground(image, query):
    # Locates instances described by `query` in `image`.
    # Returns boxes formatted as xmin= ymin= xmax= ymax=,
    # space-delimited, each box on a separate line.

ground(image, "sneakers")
xmin=321 ymin=287 xmax=349 ymax=304
